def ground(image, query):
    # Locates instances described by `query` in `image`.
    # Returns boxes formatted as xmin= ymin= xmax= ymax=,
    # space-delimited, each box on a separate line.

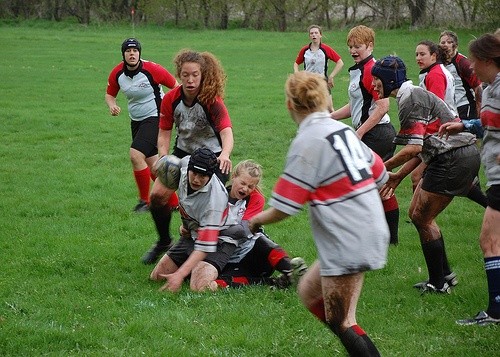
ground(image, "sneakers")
xmin=291 ymin=257 xmax=308 ymax=281
xmin=142 ymin=238 xmax=173 ymax=264
xmin=421 ymin=283 xmax=451 ymax=296
xmin=270 ymin=274 xmax=291 ymax=289
xmin=414 ymin=272 xmax=458 ymax=287
xmin=134 ymin=201 xmax=150 ymax=211
xmin=456 ymin=310 xmax=500 ymax=326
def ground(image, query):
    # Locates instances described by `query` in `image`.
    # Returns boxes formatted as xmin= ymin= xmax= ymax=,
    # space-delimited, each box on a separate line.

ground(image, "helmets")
xmin=121 ymin=38 xmax=141 ymax=53
xmin=371 ymin=57 xmax=407 ymax=98
xmin=188 ymin=148 xmax=218 ymax=175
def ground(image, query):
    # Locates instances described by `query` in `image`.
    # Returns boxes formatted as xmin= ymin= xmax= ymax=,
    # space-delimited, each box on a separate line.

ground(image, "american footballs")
xmin=158 ymin=154 xmax=182 ymax=189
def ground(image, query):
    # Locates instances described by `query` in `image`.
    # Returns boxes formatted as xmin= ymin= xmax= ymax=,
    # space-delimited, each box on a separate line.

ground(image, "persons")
xmin=410 ymin=40 xmax=456 ymax=192
xmin=150 ymin=145 xmax=251 ymax=293
xmin=438 ymin=30 xmax=484 ymax=120
xmin=294 ymin=25 xmax=344 ymax=114
xmin=142 ymin=47 xmax=234 ymax=264
xmin=330 ymin=25 xmax=400 ymax=246
xmin=246 ymin=71 xmax=392 ymax=356
xmin=180 ymin=157 xmax=309 ymax=293
xmin=105 ymin=37 xmax=180 ymax=214
xmin=455 ymin=32 xmax=500 ymax=327
xmin=369 ymin=56 xmax=480 ymax=299
